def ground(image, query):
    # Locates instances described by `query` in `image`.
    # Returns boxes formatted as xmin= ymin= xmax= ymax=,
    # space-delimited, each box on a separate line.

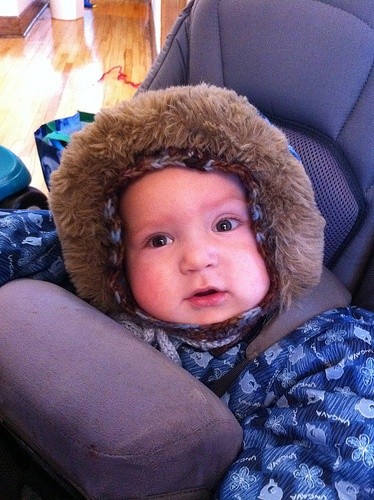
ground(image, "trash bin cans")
xmin=50 ymin=0 xmax=84 ymax=21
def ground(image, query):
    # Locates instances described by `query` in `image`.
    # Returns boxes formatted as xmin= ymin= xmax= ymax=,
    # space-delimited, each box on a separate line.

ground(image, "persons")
xmin=0 ymin=82 xmax=374 ymax=500
